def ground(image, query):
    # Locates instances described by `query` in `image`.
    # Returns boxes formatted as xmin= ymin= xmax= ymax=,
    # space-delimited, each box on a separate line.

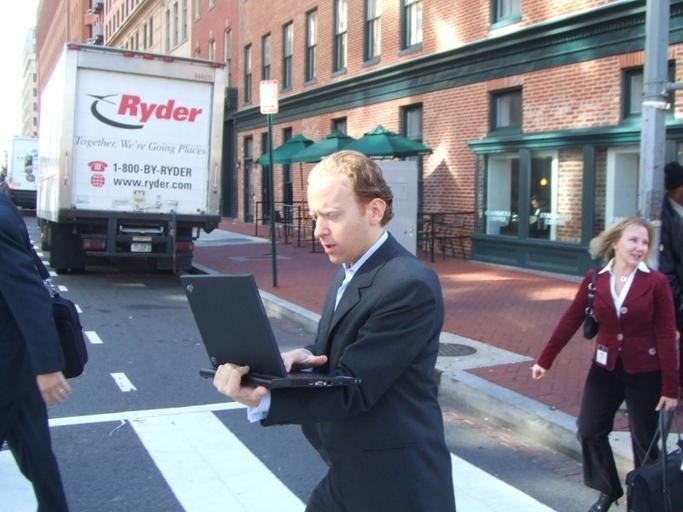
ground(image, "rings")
xmin=59 ymin=389 xmax=64 ymax=394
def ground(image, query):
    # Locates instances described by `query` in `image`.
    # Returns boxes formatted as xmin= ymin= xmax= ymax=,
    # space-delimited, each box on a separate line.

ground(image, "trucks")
xmin=34 ymin=41 xmax=226 ymax=278
xmin=4 ymin=133 xmax=37 ymax=213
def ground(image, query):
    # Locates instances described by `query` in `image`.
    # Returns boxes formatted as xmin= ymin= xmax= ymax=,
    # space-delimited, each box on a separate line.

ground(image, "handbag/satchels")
xmin=624 ymin=449 xmax=683 ymax=512
xmin=51 ymin=296 xmax=88 ymax=379
xmin=582 ymin=314 xmax=599 ymax=339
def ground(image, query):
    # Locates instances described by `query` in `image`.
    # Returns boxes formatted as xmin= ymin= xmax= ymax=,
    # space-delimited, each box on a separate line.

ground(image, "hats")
xmin=664 ymin=161 xmax=683 ymax=190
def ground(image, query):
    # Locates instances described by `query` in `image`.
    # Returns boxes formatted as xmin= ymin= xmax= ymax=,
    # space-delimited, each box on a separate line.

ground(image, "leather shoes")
xmin=587 ymin=490 xmax=624 ymax=512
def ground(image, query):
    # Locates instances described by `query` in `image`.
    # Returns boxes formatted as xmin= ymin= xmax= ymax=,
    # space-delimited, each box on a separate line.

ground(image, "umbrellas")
xmin=343 ymin=124 xmax=432 ymax=160
xmin=291 ymin=130 xmax=358 ymax=162
xmin=255 ymin=133 xmax=315 ymax=239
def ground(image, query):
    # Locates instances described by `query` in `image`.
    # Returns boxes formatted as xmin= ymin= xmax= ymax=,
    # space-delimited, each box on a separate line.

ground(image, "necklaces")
xmin=621 ymin=272 xmax=628 ymax=282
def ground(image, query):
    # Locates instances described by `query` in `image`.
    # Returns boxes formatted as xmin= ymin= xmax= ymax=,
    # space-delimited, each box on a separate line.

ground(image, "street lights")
xmin=258 ymin=79 xmax=281 ymax=289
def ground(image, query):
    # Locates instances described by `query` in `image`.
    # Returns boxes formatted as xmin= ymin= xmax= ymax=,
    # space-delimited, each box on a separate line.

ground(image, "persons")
xmin=212 ymin=148 xmax=456 ymax=511
xmin=530 ymin=195 xmax=546 ymax=237
xmin=659 ymin=161 xmax=683 ymax=336
xmin=531 ymin=216 xmax=682 ymax=512
xmin=0 ymin=191 xmax=71 ymax=511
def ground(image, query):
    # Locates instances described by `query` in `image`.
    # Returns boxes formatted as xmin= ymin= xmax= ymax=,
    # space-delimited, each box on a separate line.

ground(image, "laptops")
xmin=181 ymin=275 xmax=362 ymax=387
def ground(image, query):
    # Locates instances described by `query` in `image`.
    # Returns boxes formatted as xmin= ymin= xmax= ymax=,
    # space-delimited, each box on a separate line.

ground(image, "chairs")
xmin=252 ymin=199 xmax=474 ymax=264
